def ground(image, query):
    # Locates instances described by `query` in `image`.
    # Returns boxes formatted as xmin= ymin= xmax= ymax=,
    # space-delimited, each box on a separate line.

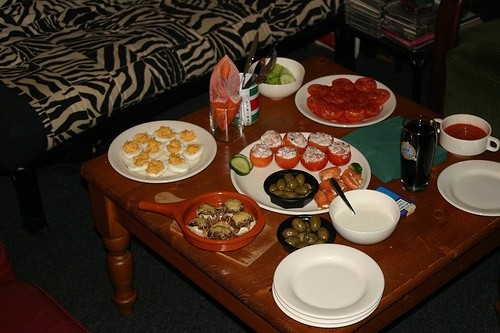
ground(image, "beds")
xmin=0 ymin=0 xmax=344 ymax=235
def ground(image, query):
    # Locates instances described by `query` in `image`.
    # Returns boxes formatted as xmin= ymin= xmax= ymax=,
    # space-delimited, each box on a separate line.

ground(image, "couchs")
xmin=0 ymin=241 xmax=89 ymax=333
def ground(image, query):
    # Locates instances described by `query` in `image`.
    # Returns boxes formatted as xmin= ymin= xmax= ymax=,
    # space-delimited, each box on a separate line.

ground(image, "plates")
xmin=271 ymin=244 xmax=385 ymax=328
xmin=230 ymin=132 xmax=371 ymax=214
xmin=107 ymin=120 xmax=217 ymax=182
xmin=437 ymin=160 xmax=500 ymax=217
xmin=294 ymin=74 xmax=396 ymax=127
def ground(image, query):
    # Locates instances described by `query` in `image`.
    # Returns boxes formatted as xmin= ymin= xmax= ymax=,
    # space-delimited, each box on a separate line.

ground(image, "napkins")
xmin=339 ymin=115 xmax=447 ymax=184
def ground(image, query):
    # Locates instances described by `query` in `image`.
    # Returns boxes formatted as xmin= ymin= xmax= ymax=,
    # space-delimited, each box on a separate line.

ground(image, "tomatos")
xmin=307 ymin=78 xmax=390 ymax=123
xmin=249 ymin=130 xmax=352 ymax=170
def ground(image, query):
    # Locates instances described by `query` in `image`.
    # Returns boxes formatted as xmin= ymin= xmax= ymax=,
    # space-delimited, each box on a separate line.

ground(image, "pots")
xmin=138 ymin=192 xmax=266 ymax=252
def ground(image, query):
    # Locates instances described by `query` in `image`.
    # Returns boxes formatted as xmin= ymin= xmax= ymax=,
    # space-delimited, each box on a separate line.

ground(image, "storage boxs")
xmin=375 ymin=187 xmax=416 ymax=218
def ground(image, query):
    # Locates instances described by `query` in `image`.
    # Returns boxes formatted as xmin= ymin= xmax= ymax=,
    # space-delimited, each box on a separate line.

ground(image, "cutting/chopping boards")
xmin=154 ymin=192 xmax=278 ymax=267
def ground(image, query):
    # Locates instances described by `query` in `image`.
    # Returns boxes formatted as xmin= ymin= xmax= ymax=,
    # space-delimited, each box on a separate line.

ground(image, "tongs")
xmin=242 ymin=31 xmax=283 ymax=89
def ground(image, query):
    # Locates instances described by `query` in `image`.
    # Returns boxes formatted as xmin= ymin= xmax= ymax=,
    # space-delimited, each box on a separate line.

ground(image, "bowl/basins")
xmin=277 ymin=215 xmax=336 ymax=252
xmin=263 ymin=169 xmax=319 ymax=209
xmin=249 ymin=57 xmax=305 ymax=101
xmin=329 ymin=189 xmax=400 ymax=245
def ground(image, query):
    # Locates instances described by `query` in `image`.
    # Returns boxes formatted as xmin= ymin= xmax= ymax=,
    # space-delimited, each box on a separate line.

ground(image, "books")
xmin=344 ymin=0 xmax=483 ymax=52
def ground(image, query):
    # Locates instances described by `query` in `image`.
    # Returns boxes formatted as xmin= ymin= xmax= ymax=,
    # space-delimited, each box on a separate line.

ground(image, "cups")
xmin=433 ymin=113 xmax=500 ymax=156
xmin=400 ymin=120 xmax=438 ymax=192
xmin=237 ymin=72 xmax=260 ymax=126
xmin=209 ymin=102 xmax=243 ymax=144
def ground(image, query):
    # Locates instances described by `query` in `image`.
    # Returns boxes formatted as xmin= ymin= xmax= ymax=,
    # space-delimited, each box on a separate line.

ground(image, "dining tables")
xmin=81 ymin=56 xmax=500 ymax=333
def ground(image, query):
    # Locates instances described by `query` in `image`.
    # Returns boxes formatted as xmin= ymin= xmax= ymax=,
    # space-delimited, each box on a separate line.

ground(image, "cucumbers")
xmin=349 ymin=162 xmax=363 ymax=173
xmin=266 ymin=63 xmax=297 ymax=84
xmin=229 ymin=154 xmax=253 ymax=175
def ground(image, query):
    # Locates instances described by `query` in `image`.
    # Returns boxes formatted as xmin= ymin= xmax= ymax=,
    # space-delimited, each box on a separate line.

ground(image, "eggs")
xmin=121 ymin=126 xmax=203 ymax=177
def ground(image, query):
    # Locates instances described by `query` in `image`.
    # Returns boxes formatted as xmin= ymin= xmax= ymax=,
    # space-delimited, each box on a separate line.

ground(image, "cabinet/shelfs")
xmin=334 ymin=0 xmax=464 ymax=113
